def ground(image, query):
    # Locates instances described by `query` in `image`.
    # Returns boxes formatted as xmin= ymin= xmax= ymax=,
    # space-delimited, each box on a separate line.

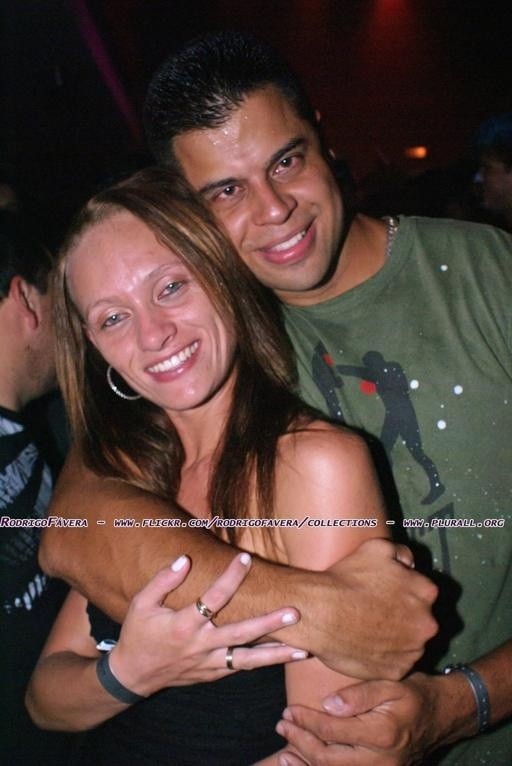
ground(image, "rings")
xmin=226 ymin=648 xmax=235 ymax=670
xmin=195 ymin=598 xmax=215 ymax=618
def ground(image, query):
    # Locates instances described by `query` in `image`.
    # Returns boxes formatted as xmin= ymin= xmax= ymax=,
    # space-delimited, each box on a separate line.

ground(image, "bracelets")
xmin=443 ymin=663 xmax=491 ymax=735
xmin=96 ymin=653 xmax=149 ymax=705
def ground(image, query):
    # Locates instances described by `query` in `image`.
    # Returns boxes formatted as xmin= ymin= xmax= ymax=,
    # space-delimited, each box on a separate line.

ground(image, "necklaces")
xmin=380 ymin=216 xmax=398 ymax=260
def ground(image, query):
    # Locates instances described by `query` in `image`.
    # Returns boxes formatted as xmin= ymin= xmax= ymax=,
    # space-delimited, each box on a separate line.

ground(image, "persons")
xmin=37 ymin=29 xmax=512 ymax=766
xmin=0 ymin=202 xmax=72 ymax=766
xmin=351 ymin=110 xmax=512 ymax=234
xmin=24 ymin=163 xmax=399 ymax=766
